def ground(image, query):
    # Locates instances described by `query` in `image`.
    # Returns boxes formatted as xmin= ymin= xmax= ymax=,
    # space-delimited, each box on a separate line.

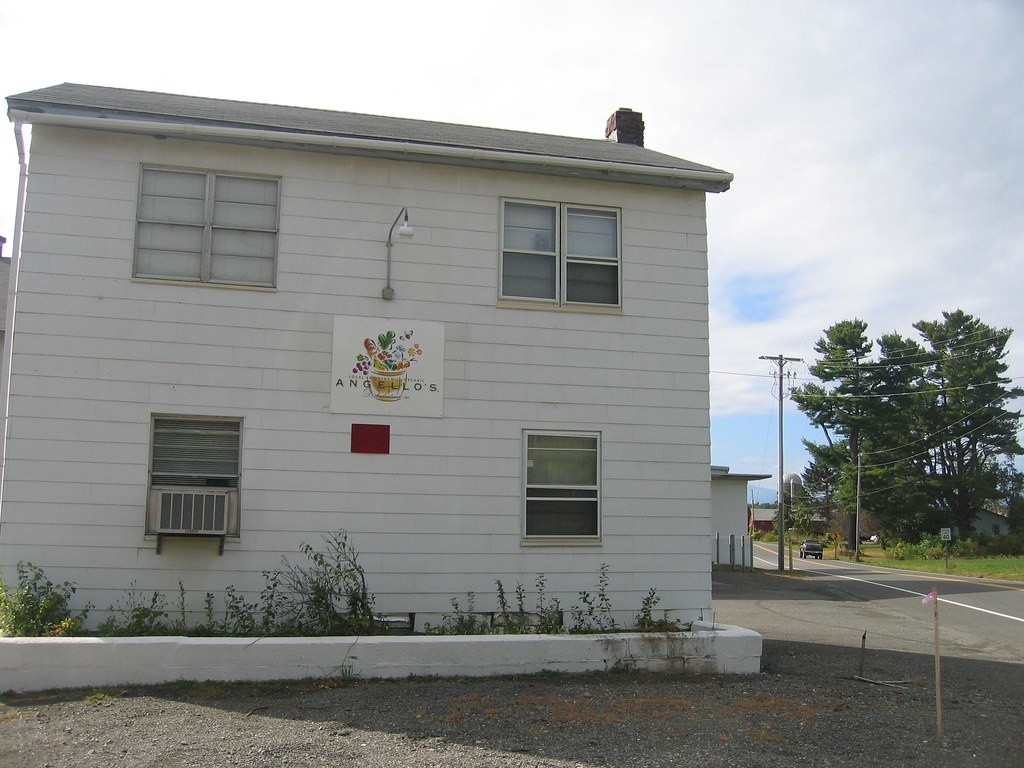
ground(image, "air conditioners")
xmin=147 ymin=485 xmax=240 ymax=538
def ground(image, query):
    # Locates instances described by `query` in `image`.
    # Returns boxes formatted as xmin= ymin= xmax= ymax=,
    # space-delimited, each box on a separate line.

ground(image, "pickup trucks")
xmin=799 ymin=539 xmax=823 ymax=560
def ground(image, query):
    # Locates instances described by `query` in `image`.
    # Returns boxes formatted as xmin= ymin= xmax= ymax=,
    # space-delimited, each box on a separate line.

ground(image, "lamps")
xmin=380 ymin=205 xmax=415 ymax=303
